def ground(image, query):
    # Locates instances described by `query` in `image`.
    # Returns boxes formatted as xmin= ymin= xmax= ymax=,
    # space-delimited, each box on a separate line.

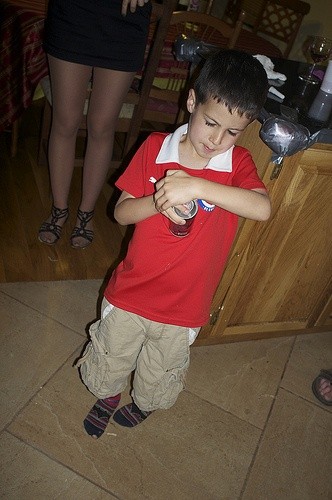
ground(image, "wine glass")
xmin=298 ymin=36 xmax=332 ymax=84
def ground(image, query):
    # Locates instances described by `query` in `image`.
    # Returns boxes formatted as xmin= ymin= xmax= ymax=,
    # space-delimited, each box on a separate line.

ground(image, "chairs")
xmin=36 ymin=0 xmax=247 ymax=173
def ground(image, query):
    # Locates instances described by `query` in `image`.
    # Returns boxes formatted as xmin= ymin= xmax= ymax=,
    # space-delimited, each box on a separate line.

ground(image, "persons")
xmin=38 ymin=0 xmax=151 ymax=248
xmin=311 ymin=367 xmax=332 ymax=407
xmin=78 ymin=47 xmax=271 ymax=438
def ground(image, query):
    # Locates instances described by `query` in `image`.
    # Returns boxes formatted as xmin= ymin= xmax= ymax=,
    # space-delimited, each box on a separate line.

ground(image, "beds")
xmin=0 ymin=0 xmax=310 ymax=159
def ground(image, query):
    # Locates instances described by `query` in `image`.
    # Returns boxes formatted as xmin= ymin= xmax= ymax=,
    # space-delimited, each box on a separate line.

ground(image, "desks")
xmin=191 ymin=58 xmax=332 ymax=348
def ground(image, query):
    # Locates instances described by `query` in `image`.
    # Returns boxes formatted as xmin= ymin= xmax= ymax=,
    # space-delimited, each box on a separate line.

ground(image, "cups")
xmin=320 ymin=60 xmax=332 ymax=94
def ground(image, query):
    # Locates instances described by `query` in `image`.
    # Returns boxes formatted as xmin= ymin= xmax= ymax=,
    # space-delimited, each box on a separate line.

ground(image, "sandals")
xmin=311 ymin=369 xmax=332 ymax=406
xmin=37 ymin=200 xmax=69 ymax=245
xmin=70 ymin=208 xmax=96 ymax=249
